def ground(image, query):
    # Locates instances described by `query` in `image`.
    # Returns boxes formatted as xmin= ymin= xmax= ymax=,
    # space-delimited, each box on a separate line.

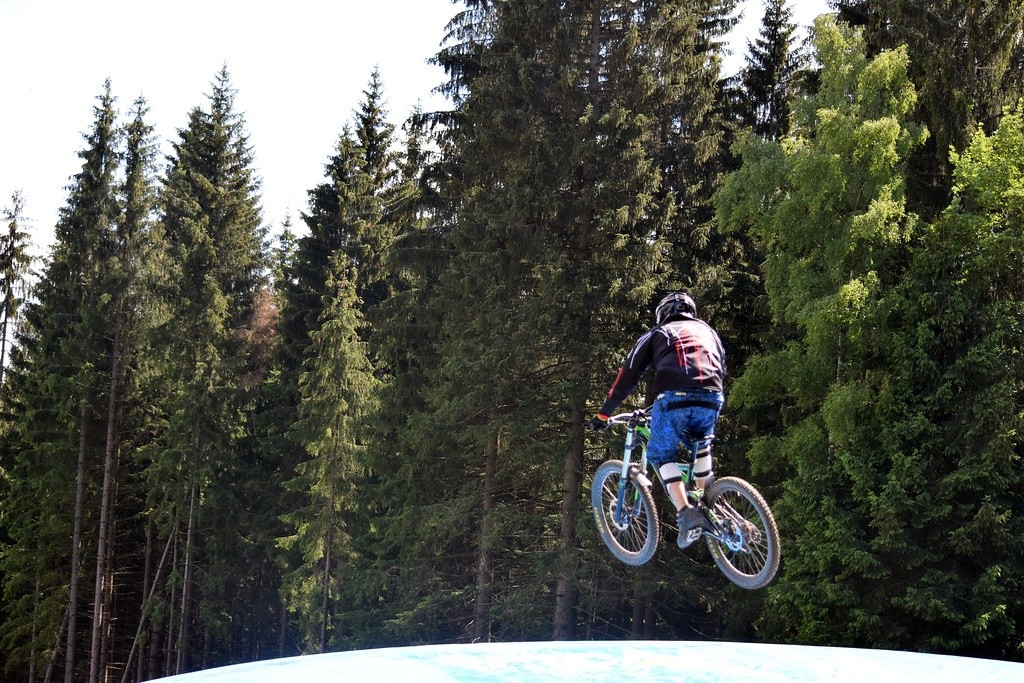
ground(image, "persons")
xmin=590 ymin=291 xmax=725 ymax=548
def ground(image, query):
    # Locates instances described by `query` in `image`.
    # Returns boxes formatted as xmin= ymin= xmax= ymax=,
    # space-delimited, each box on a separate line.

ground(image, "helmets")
xmin=656 ymin=292 xmax=697 ymax=325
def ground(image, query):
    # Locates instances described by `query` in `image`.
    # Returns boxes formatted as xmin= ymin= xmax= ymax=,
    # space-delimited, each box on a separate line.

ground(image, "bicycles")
xmin=591 ymin=402 xmax=782 ymax=590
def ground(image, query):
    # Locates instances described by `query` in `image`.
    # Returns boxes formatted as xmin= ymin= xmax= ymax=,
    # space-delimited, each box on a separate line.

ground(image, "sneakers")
xmin=676 ymin=505 xmax=704 ymax=548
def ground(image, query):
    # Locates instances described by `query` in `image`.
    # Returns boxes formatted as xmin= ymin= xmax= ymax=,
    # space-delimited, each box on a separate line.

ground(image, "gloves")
xmin=589 ymin=416 xmax=607 ymax=432
